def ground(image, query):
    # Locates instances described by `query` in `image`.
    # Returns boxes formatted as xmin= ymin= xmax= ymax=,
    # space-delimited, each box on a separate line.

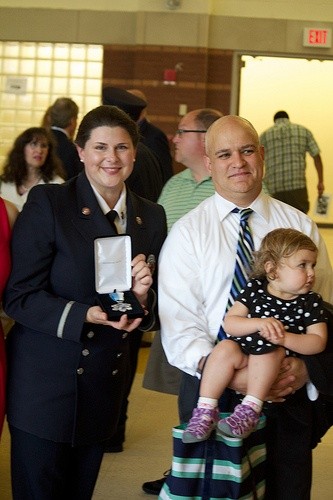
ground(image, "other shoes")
xmin=143 ymin=468 xmax=171 ymax=495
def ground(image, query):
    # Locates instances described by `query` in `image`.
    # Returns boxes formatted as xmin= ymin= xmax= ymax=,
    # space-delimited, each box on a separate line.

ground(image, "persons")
xmin=0 ymin=199 xmax=19 ymax=436
xmin=157 ymin=109 xmax=269 ymax=233
xmin=0 ymin=106 xmax=168 ymax=500
xmin=44 ymin=97 xmax=85 ymax=181
xmin=102 ymin=86 xmax=163 ymax=202
xmin=259 ymin=110 xmax=324 ymax=214
xmin=183 ymin=228 xmax=329 ymax=440
xmin=157 ymin=115 xmax=333 ymax=500
xmin=129 ymin=89 xmax=174 ymax=183
xmin=0 ymin=127 xmax=65 ymax=212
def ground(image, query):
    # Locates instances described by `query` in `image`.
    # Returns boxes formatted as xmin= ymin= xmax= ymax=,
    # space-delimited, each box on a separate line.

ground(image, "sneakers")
xmin=182 ymin=407 xmax=219 ymax=443
xmin=217 ymin=404 xmax=260 ymax=439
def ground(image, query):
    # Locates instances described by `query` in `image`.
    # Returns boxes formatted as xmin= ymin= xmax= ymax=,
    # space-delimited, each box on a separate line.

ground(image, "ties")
xmin=214 ymin=207 xmax=256 ymax=346
xmin=104 ymin=210 xmax=119 ymax=236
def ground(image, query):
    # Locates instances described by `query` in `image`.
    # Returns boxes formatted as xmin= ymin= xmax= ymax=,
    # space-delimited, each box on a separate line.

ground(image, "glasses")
xmin=176 ymin=130 xmax=207 ymax=137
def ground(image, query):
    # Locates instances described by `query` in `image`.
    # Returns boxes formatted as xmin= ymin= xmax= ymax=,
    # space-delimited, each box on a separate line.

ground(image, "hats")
xmin=103 ymin=87 xmax=147 ymax=120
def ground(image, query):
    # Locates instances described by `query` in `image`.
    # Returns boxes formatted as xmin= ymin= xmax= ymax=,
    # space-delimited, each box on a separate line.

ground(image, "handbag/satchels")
xmin=158 ymin=413 xmax=266 ymax=500
